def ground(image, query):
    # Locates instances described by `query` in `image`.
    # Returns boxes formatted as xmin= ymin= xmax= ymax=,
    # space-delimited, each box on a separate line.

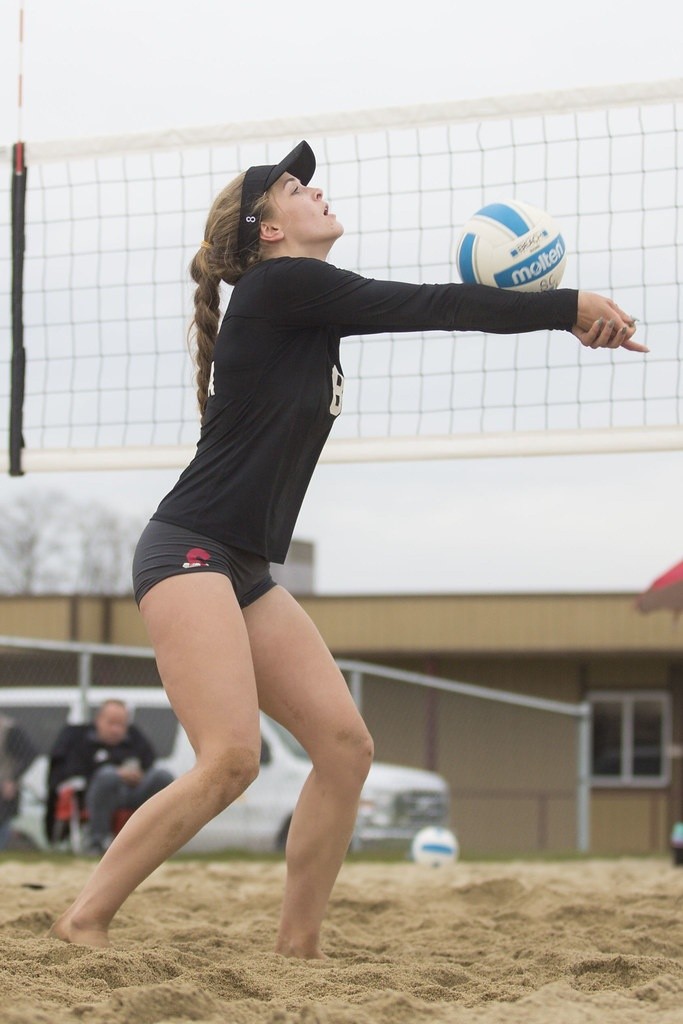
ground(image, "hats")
xmin=236 ymin=141 xmax=315 ymax=270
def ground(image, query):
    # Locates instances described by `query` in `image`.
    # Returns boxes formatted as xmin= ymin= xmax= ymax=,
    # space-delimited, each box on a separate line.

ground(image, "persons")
xmin=1 ymin=701 xmax=174 ymax=858
xmin=46 ymin=142 xmax=649 ymax=955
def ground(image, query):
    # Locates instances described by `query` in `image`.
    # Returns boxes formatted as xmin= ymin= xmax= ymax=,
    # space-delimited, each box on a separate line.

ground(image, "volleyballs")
xmin=456 ymin=197 xmax=567 ymax=293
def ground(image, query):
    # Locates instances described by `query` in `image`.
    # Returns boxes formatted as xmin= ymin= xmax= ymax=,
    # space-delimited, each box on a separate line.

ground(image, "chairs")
xmin=51 ymin=725 xmax=136 ymax=853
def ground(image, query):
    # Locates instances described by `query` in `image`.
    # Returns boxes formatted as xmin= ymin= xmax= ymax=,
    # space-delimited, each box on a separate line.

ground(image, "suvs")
xmin=1 ymin=688 xmax=448 ymax=854
